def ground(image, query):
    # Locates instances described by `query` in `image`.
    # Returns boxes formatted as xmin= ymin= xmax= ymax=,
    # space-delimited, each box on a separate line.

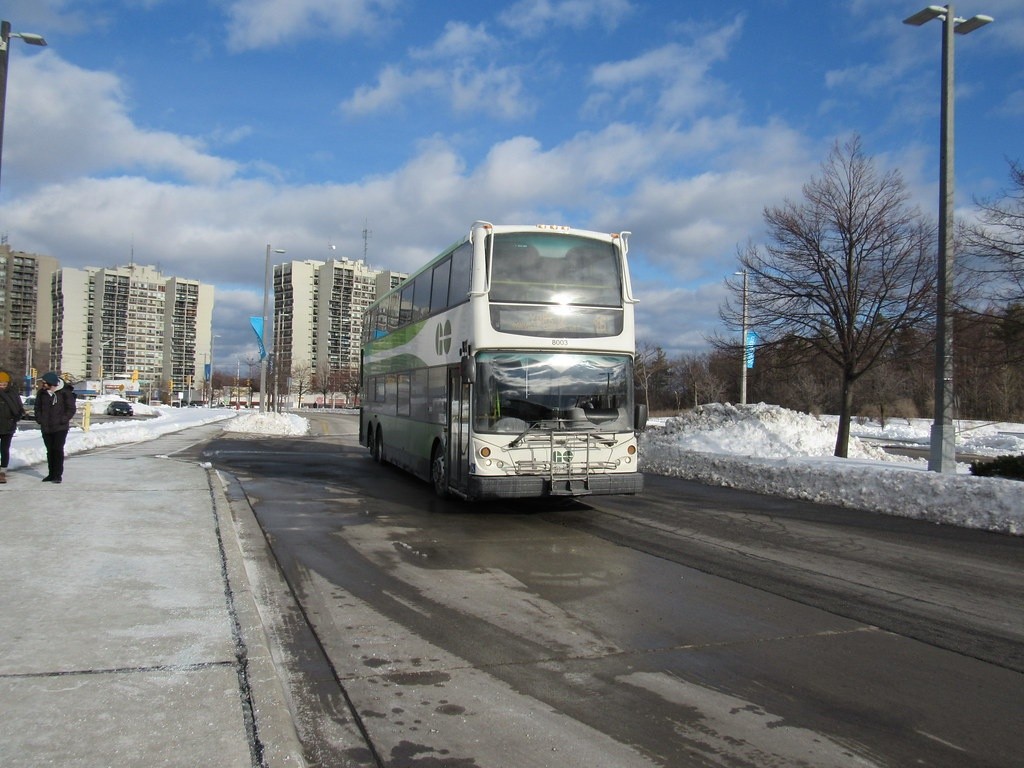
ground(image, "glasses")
xmin=42 ymin=382 xmax=46 ymax=384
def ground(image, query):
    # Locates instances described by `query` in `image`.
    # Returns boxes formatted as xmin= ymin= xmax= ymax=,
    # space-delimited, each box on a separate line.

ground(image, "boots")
xmin=0 ymin=467 xmax=7 ymax=482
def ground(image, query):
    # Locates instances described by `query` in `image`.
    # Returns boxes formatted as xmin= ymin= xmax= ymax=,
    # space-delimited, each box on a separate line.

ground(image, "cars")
xmin=21 ymin=398 xmax=38 ymax=420
xmin=108 ymin=401 xmax=134 ymax=417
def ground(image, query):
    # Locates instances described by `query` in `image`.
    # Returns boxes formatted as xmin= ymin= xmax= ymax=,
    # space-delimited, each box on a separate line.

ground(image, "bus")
xmin=358 ymin=218 xmax=649 ymax=503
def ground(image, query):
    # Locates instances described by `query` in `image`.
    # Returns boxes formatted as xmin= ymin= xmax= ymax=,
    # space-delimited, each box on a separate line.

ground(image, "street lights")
xmin=732 ymin=268 xmax=750 ymax=411
xmin=272 ymin=312 xmax=291 ymax=413
xmin=100 ymin=335 xmax=126 ymax=396
xmin=208 ymin=334 xmax=223 ymax=410
xmin=257 ymin=245 xmax=286 ymax=415
xmin=0 ymin=20 xmax=47 ymax=180
xmin=901 ymin=5 xmax=996 ymax=474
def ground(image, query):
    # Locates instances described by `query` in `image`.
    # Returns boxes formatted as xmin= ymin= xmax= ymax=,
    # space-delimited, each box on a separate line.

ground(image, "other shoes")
xmin=42 ymin=474 xmax=62 ymax=483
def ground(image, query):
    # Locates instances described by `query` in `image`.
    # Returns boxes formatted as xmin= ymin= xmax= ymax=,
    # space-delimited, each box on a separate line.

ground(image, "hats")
xmin=0 ymin=372 xmax=9 ymax=383
xmin=42 ymin=372 xmax=58 ymax=386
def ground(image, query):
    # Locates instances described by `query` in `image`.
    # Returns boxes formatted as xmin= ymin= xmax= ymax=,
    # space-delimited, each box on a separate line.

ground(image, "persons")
xmin=0 ymin=370 xmax=24 ymax=483
xmin=570 ymin=383 xmax=602 ymax=410
xmin=34 ymin=372 xmax=75 ymax=483
